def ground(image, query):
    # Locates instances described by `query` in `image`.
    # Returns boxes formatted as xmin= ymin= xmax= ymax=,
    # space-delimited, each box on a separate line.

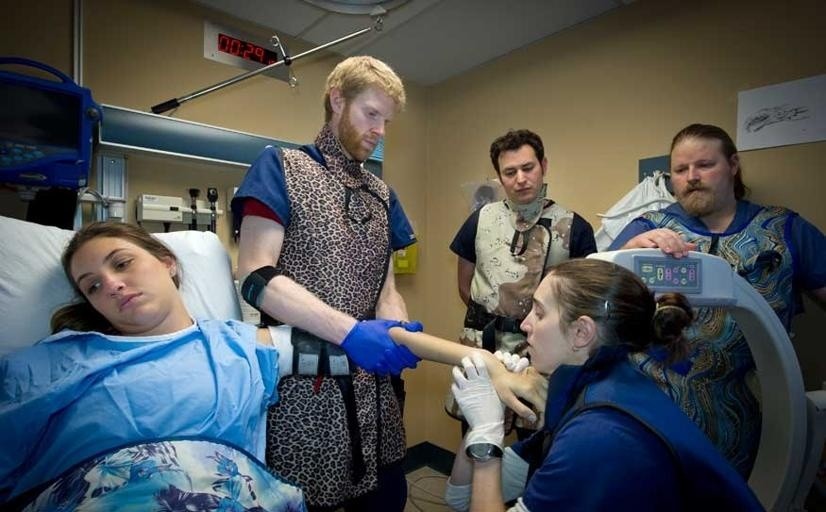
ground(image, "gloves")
xmin=450 ymin=349 xmax=529 ymax=455
xmin=337 ymin=317 xmax=423 ymax=379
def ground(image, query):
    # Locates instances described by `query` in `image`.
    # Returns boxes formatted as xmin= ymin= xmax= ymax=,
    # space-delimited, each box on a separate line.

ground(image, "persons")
xmin=606 ymin=123 xmax=826 ymax=339
xmin=232 ymin=55 xmax=423 ymax=512
xmin=443 ymin=260 xmax=767 ymax=511
xmin=0 ymin=220 xmax=551 ymax=512
xmin=449 ymin=129 xmax=597 ymax=447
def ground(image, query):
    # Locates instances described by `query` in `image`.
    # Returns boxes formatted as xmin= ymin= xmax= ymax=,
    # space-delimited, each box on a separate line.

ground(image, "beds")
xmin=1 ymin=216 xmax=243 ymax=355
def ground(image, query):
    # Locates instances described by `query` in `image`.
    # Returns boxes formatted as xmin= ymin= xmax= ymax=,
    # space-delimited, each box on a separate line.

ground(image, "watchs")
xmin=465 ymin=437 xmax=503 ymax=460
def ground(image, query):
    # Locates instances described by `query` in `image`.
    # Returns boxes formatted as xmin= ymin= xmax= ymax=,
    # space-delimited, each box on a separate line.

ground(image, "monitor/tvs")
xmin=0 ymin=56 xmax=104 ymax=188
